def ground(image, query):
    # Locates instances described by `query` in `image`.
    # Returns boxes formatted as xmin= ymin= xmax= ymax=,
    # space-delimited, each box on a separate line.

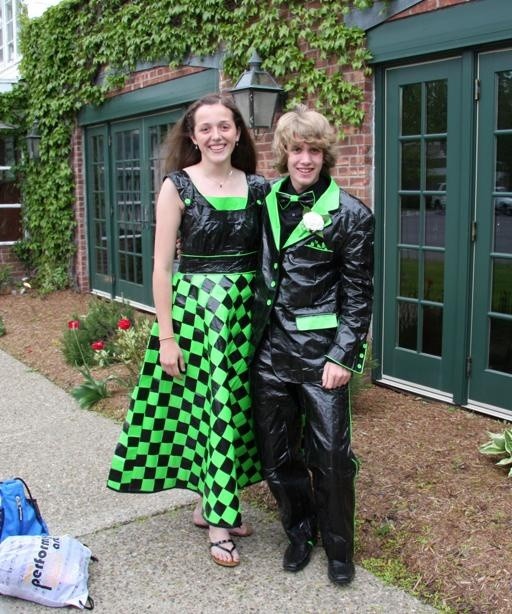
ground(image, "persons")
xmin=103 ymin=93 xmax=271 ymax=568
xmin=175 ymin=106 xmax=375 ymax=585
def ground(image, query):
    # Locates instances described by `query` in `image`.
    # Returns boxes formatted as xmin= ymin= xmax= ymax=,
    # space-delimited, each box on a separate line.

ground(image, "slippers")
xmin=209 ymin=539 xmax=239 ymax=567
xmin=194 ymin=523 xmax=254 ymax=537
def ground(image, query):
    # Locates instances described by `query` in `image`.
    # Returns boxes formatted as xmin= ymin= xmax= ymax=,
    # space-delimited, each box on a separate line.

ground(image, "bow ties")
xmin=276 ymin=190 xmax=315 ymax=211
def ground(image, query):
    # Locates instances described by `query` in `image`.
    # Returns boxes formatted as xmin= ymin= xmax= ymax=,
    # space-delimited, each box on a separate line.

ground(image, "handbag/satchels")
xmin=0 ymin=477 xmax=99 ymax=611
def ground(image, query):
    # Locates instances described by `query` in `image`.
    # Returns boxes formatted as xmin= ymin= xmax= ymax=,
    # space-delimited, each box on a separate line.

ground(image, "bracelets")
xmin=158 ymin=336 xmax=176 ymax=342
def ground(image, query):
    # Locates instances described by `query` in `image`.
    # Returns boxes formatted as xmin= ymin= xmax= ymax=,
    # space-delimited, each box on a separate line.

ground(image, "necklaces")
xmin=197 ymin=167 xmax=234 ymax=189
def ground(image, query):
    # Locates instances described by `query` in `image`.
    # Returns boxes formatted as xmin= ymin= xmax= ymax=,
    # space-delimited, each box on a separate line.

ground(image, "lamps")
xmin=20 ymin=118 xmax=44 ymax=160
xmin=227 ymin=47 xmax=291 ymax=138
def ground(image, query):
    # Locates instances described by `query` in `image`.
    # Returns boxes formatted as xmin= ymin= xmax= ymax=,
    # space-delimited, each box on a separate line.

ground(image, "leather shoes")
xmin=327 ymin=557 xmax=355 ymax=584
xmin=283 ymin=538 xmax=317 ymax=572
